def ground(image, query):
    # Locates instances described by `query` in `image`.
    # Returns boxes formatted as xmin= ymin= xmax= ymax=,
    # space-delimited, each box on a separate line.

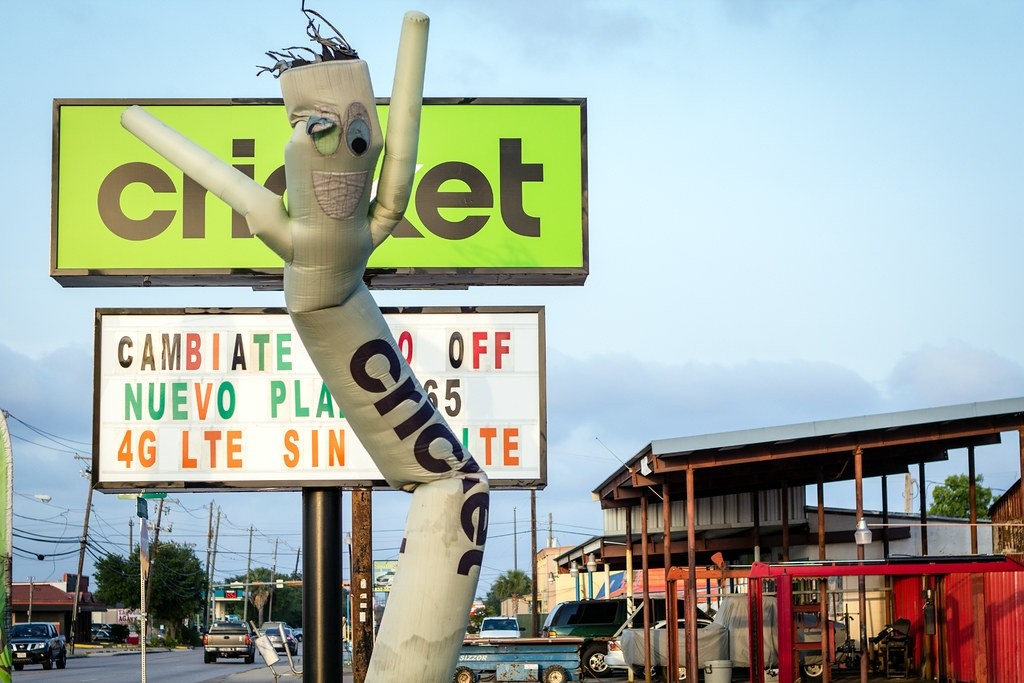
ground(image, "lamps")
xmin=547 ymin=572 xmax=559 ymax=585
xmin=587 ymin=553 xmax=597 ymax=572
xmin=570 ymin=560 xmax=579 ymax=578
xmin=855 ymin=517 xmax=872 ymax=544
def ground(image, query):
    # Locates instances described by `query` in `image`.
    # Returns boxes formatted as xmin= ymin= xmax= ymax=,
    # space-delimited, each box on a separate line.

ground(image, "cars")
xmin=603 ymin=618 xmax=728 ymax=683
xmin=262 ymin=627 xmax=298 ymax=656
xmin=257 ymin=621 xmax=304 ymax=642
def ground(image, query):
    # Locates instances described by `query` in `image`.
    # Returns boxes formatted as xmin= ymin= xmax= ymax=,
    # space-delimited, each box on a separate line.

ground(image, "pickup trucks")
xmin=203 ymin=620 xmax=258 ymax=664
xmin=10 ymin=622 xmax=66 ymax=671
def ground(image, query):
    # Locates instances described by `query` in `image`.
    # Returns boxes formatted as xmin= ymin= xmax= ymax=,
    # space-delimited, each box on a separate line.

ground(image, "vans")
xmin=473 ymin=615 xmax=526 ymax=639
xmin=539 ymin=597 xmax=714 ymax=678
